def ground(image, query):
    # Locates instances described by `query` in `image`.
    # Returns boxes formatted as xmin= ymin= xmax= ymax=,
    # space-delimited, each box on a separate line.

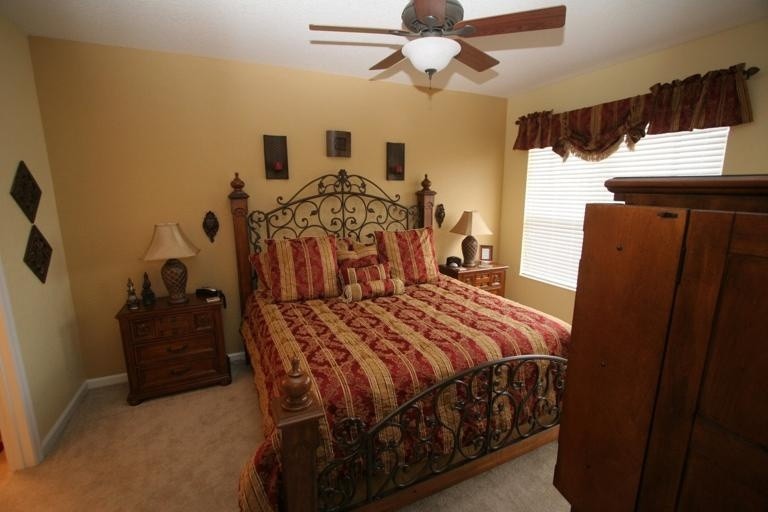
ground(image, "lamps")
xmin=398 ymin=32 xmax=462 ymax=96
xmin=446 ymin=209 xmax=495 ymax=267
xmin=139 ymin=220 xmax=203 ymax=305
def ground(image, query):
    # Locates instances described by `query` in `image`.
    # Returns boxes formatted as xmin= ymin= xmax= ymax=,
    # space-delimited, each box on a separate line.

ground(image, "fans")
xmin=305 ymin=0 xmax=569 ymax=76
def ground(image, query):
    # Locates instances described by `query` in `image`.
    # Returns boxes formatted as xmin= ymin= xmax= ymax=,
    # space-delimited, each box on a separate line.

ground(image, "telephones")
xmin=196 ymin=286 xmax=218 ymax=297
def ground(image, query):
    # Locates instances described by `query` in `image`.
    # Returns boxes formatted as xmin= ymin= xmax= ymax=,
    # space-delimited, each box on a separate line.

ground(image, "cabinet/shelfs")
xmin=546 ymin=167 xmax=768 ymax=512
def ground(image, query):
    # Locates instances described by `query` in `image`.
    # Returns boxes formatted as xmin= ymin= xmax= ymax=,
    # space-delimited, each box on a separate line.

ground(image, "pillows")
xmin=244 ymin=222 xmax=440 ymax=304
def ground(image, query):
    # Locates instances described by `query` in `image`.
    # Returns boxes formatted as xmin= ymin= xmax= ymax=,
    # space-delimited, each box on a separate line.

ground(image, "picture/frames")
xmin=478 ymin=243 xmax=495 ymax=266
xmin=325 ymin=129 xmax=351 ymax=160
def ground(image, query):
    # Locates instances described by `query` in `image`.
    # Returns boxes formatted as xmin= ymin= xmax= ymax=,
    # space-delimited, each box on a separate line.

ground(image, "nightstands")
xmin=437 ymin=258 xmax=511 ymax=301
xmin=111 ymin=285 xmax=235 ymax=407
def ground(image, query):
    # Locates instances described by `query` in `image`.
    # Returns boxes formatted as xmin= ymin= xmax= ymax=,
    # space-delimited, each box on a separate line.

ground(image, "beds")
xmin=224 ymin=165 xmax=578 ymax=511
